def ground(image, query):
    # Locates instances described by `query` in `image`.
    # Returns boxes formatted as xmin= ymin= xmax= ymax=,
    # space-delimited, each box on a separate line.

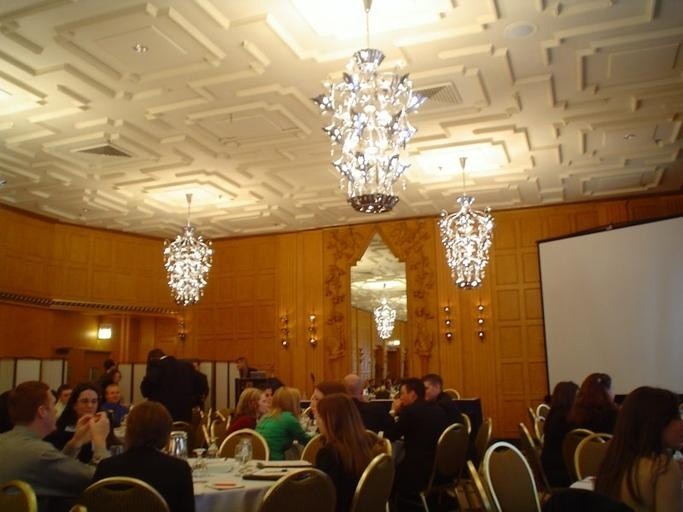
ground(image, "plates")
xmin=205 ymin=482 xmax=245 ymax=491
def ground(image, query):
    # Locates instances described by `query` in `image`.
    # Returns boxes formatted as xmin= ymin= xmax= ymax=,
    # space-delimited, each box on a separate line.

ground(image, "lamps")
xmin=309 ymin=0 xmax=427 ymax=217
xmin=161 ymin=193 xmax=214 ymax=303
xmin=95 ymin=319 xmax=114 ymax=340
xmin=370 ymin=283 xmax=396 ymax=341
xmin=439 ymin=159 xmax=495 ymax=288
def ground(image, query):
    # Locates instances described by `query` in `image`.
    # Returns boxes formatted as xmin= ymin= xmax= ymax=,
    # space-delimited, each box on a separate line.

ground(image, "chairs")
xmin=572 ymin=433 xmax=614 ymax=481
xmin=466 ymin=459 xmax=492 ymax=510
xmin=300 ymin=433 xmax=325 ymax=468
xmin=483 ymin=442 xmax=542 ymax=511
xmin=2 ymin=477 xmax=38 ymax=511
xmin=411 ymin=422 xmax=477 ymax=511
xmin=216 ymin=427 xmax=271 ymax=462
xmin=78 ymin=476 xmax=171 ymax=510
xmin=562 ymin=428 xmax=607 ymax=479
xmin=363 ymin=388 xmax=553 ymax=454
xmin=348 ymin=453 xmax=395 ymax=511
xmin=256 ymin=467 xmax=338 ymax=511
xmin=127 ymin=404 xmax=234 ymax=455
xmin=518 ymin=423 xmax=577 ymax=511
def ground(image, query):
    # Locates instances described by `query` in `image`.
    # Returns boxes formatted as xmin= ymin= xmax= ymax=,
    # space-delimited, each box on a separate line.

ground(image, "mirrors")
xmin=349 ymin=230 xmax=408 ymax=397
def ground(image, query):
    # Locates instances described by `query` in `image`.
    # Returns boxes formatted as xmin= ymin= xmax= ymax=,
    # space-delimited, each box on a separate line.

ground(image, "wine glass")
xmin=190 ymin=448 xmax=209 ymax=483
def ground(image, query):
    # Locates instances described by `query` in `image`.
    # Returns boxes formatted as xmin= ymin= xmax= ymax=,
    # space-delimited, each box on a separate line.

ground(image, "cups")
xmin=232 ymin=438 xmax=253 ymax=466
xmin=303 ymin=407 xmax=313 ymax=419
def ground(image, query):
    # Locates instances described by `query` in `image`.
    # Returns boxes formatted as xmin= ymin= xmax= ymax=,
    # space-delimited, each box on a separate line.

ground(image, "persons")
xmin=595 ymin=386 xmax=681 ymax=511
xmin=219 ymin=357 xmax=466 ymax=511
xmin=569 ymin=371 xmax=619 ymax=442
xmin=538 ymin=381 xmax=578 ymax=483
xmin=1 ymin=349 xmax=209 ymax=512
